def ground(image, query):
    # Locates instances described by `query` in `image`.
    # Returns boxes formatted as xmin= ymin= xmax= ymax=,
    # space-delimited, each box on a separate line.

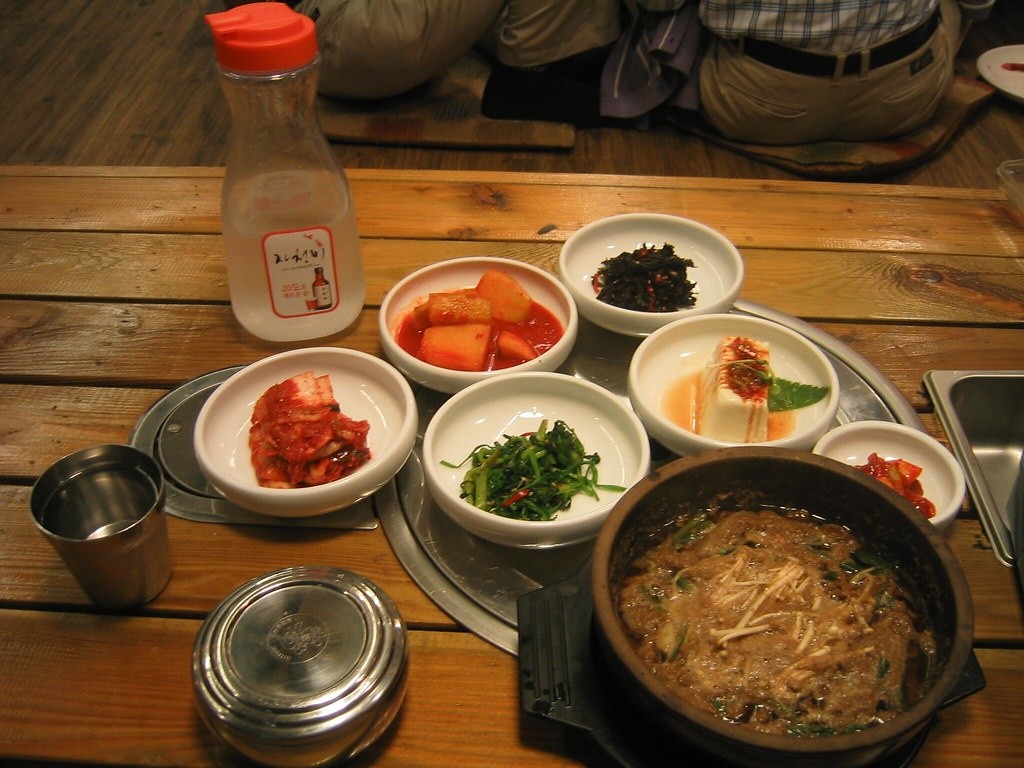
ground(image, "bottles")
xmin=313 ymin=267 xmax=332 ymax=309
xmin=205 ymin=3 xmax=365 ymax=343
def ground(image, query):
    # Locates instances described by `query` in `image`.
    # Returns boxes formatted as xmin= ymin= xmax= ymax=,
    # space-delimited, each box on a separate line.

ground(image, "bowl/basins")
xmin=195 ymin=347 xmax=419 ymax=519
xmin=560 ymin=213 xmax=744 ymax=338
xmin=628 ymin=314 xmax=839 ymax=459
xmin=811 ymin=421 xmax=965 ymax=529
xmin=378 ymin=257 xmax=577 ymax=393
xmin=423 ymin=371 xmax=650 ymax=548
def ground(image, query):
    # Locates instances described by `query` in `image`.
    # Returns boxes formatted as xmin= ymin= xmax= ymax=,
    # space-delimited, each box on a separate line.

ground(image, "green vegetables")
xmin=736 ymin=361 xmax=829 ymax=413
xmin=439 ymin=419 xmax=630 ymax=522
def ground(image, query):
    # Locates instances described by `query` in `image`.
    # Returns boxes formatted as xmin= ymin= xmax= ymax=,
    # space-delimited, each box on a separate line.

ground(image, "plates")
xmin=978 ymin=44 xmax=1024 ymax=103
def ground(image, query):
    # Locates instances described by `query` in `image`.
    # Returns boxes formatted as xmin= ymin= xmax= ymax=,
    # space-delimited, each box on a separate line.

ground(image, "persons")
xmin=293 ymin=0 xmax=997 ymax=148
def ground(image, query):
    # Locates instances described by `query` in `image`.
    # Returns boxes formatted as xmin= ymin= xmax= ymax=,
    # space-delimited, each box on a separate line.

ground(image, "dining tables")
xmin=0 ymin=164 xmax=1024 ymax=768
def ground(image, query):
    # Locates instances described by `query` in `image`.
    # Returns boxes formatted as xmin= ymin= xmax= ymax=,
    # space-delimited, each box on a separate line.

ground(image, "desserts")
xmin=693 ymin=335 xmax=772 ymax=444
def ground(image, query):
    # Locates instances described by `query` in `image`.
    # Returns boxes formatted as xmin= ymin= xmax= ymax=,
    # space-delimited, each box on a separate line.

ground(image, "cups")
xmin=28 ymin=444 xmax=171 ymax=608
xmin=305 ymin=297 xmax=317 ymax=311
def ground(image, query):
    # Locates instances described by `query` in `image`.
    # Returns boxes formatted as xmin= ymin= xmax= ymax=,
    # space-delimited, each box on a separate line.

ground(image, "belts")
xmin=720 ymin=4 xmax=942 ymax=78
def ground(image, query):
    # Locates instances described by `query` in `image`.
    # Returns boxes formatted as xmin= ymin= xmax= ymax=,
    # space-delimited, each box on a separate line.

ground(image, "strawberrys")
xmin=849 ymin=453 xmax=935 ymax=519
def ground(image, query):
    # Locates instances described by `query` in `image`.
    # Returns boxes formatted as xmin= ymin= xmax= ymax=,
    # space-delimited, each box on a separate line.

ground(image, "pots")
xmin=594 ymin=446 xmax=972 ymax=768
